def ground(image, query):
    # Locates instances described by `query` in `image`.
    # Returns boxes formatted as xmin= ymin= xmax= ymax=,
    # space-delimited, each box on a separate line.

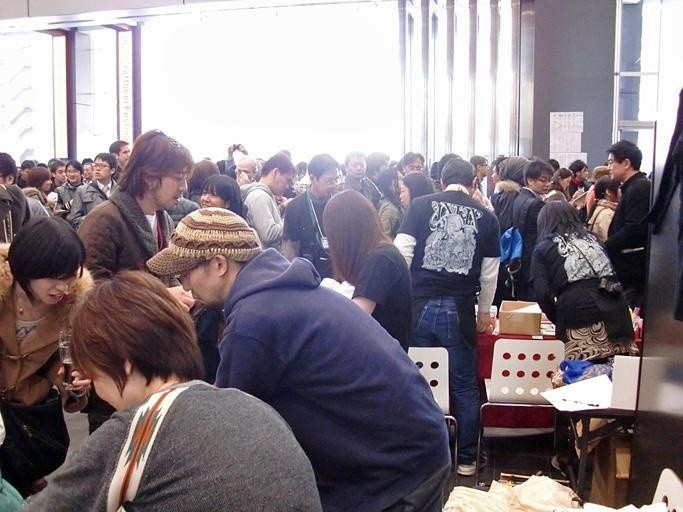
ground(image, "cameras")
xmin=598 ymin=277 xmax=624 ymax=298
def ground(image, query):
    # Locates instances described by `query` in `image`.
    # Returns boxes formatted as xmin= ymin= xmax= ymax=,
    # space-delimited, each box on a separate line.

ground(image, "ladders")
xmin=611 ymin=0 xmax=663 ymax=175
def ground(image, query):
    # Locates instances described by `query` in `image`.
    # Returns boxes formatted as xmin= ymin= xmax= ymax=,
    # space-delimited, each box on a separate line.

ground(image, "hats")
xmin=147 ymin=206 xmax=264 ymax=275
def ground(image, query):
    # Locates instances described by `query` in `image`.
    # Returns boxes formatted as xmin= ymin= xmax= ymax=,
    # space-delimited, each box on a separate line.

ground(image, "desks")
xmin=558 ymin=409 xmax=637 ymax=498
xmin=475 ymin=305 xmax=560 ymax=438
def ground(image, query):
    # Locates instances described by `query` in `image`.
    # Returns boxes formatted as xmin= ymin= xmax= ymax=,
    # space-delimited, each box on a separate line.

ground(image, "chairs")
xmin=406 ymin=346 xmax=458 ymax=482
xmin=475 ymin=339 xmax=566 ymax=467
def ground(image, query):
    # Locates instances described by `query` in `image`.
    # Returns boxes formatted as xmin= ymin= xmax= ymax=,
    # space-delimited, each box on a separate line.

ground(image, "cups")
xmin=57 ymin=328 xmax=78 ymax=389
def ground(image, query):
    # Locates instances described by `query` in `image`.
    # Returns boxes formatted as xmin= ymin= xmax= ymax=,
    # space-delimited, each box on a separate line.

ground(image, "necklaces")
xmin=12 ymin=288 xmax=42 ymax=315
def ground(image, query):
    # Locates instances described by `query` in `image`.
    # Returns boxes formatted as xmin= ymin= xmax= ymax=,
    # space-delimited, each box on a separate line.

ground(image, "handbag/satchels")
xmin=498 ymin=225 xmax=523 ymax=299
xmin=552 ymin=360 xmax=612 ymax=384
xmin=0 ymin=388 xmax=70 ymax=483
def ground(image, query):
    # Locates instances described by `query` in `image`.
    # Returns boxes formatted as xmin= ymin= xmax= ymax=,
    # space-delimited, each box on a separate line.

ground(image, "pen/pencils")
xmin=562 ymin=399 xmax=599 ymax=407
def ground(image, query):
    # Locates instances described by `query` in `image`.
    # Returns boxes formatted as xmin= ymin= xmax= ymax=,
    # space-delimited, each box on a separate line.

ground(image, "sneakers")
xmin=457 ymin=456 xmax=488 ymax=476
xmin=551 ymin=454 xmax=567 ymax=478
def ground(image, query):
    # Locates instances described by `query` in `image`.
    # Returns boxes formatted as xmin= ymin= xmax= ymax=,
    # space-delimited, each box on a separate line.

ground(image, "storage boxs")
xmin=498 ymin=299 xmax=541 ymax=337
xmin=588 ymin=434 xmax=631 ymax=510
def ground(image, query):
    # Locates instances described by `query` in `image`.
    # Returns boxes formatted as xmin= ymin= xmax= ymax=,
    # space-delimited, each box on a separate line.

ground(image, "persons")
xmin=19 ymin=272 xmax=325 ymax=512
xmin=0 ymin=215 xmax=96 ymax=499
xmin=145 ymin=207 xmax=454 ymax=512
xmin=0 ymin=469 xmax=32 ymax=512
xmin=0 ymin=130 xmax=655 ymax=476
